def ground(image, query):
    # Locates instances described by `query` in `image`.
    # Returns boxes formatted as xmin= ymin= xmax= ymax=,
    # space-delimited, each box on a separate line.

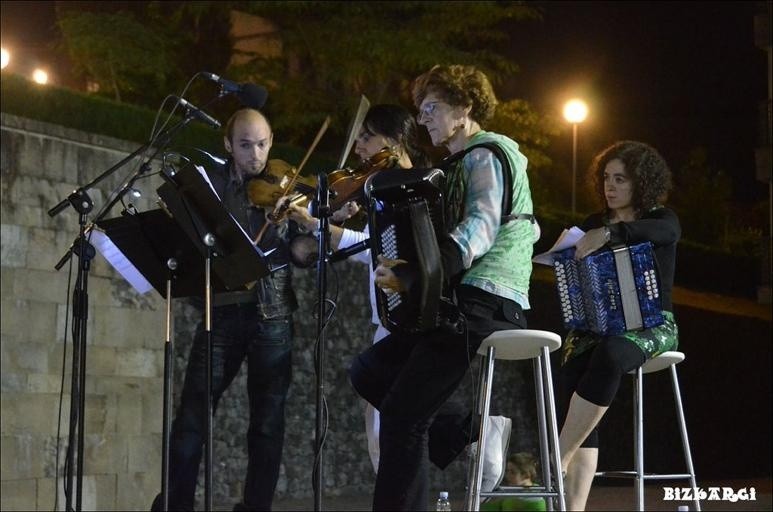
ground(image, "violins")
xmin=266 ymin=146 xmax=400 ymax=225
xmin=245 ymin=160 xmax=319 ymax=209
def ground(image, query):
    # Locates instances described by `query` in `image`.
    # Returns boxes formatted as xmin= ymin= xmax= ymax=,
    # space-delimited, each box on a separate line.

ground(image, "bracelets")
xmin=602 ymin=226 xmax=610 ymax=243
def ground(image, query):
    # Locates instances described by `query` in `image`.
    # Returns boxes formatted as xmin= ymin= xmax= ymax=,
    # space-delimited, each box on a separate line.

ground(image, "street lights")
xmin=562 ymin=98 xmax=588 ymax=225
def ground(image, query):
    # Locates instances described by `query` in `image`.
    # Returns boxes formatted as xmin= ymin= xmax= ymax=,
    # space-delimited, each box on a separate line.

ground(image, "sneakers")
xmin=465 ymin=413 xmax=514 ymax=505
xmin=534 ymin=453 xmax=568 ymax=510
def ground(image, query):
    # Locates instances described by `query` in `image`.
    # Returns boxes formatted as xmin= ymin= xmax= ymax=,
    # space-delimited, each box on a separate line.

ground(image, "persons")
xmin=478 ymin=450 xmax=547 ymax=511
xmin=349 ymin=61 xmax=545 ymax=510
xmin=271 ymin=102 xmax=431 ymax=511
xmin=545 ymin=137 xmax=682 ymax=511
xmin=146 ymin=104 xmax=363 ymax=511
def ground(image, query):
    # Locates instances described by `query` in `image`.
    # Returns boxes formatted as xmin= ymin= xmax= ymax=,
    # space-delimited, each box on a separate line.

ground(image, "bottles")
xmin=437 ymin=491 xmax=451 ymax=512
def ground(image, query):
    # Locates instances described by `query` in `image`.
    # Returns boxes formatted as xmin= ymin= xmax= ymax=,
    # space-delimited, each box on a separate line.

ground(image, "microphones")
xmin=199 ymin=71 xmax=269 ymax=111
xmin=330 ymin=237 xmax=372 ymax=264
xmin=171 ymin=94 xmax=221 ymax=131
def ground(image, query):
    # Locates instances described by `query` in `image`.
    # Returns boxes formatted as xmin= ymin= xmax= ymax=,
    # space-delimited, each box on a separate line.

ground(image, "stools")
xmin=463 ymin=328 xmax=703 ymax=512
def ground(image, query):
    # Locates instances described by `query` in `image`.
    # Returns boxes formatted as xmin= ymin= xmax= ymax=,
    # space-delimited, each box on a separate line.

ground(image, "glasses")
xmin=415 ymin=99 xmax=446 ymax=123
